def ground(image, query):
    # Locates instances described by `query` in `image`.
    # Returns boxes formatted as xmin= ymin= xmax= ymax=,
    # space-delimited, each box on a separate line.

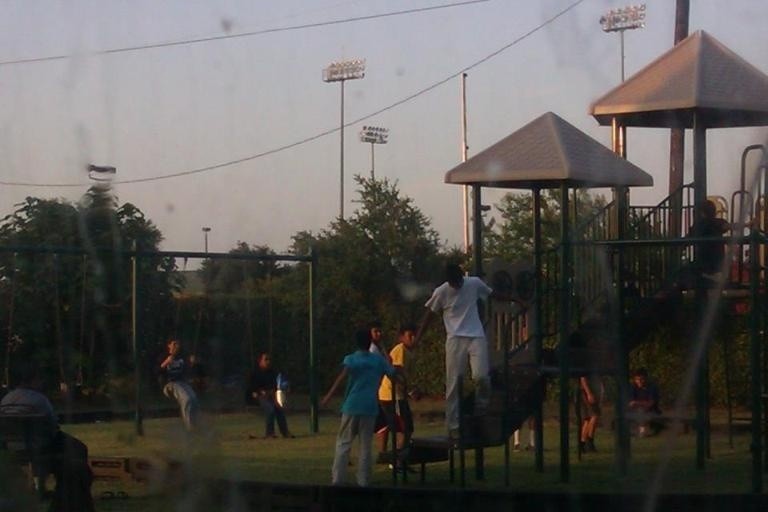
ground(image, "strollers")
xmin=1 ymin=387 xmax=95 ymax=503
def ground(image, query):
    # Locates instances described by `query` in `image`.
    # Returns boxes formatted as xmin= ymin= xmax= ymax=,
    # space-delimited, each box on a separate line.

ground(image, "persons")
xmin=680 ymin=200 xmax=732 ymax=275
xmin=414 ymin=263 xmax=532 ymax=442
xmin=627 ymin=366 xmax=662 ymax=440
xmin=0 ymin=354 xmax=61 ymax=493
xmin=159 ymin=336 xmax=204 ymax=432
xmin=320 ymin=323 xmax=416 ymax=487
xmin=514 ymin=417 xmax=537 ymax=451
xmin=244 ymin=349 xmax=295 ymax=438
xmin=577 ymin=372 xmax=601 ymax=453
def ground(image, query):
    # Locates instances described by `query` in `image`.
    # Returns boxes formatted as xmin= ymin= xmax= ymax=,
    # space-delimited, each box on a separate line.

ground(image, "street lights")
xmin=201 ymin=227 xmax=212 ymax=259
xmin=598 ymin=5 xmax=649 ymax=204
xmin=361 ymin=127 xmax=390 ymax=184
xmin=322 ymin=62 xmax=364 ymax=222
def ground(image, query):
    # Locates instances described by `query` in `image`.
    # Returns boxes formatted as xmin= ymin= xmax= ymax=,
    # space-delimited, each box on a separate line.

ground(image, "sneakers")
xmin=265 ymin=431 xmax=294 ymax=439
xmin=449 ymin=429 xmax=459 ymax=439
xmin=513 ymin=443 xmax=535 ymax=452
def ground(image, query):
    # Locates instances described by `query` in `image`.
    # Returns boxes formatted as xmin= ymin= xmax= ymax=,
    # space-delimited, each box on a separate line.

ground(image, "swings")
xmin=168 ymin=257 xmax=214 ymax=399
xmin=243 ymin=259 xmax=273 ymax=399
xmin=0 ymin=252 xmax=18 ymax=388
xmin=53 ymin=253 xmax=87 ymax=394
